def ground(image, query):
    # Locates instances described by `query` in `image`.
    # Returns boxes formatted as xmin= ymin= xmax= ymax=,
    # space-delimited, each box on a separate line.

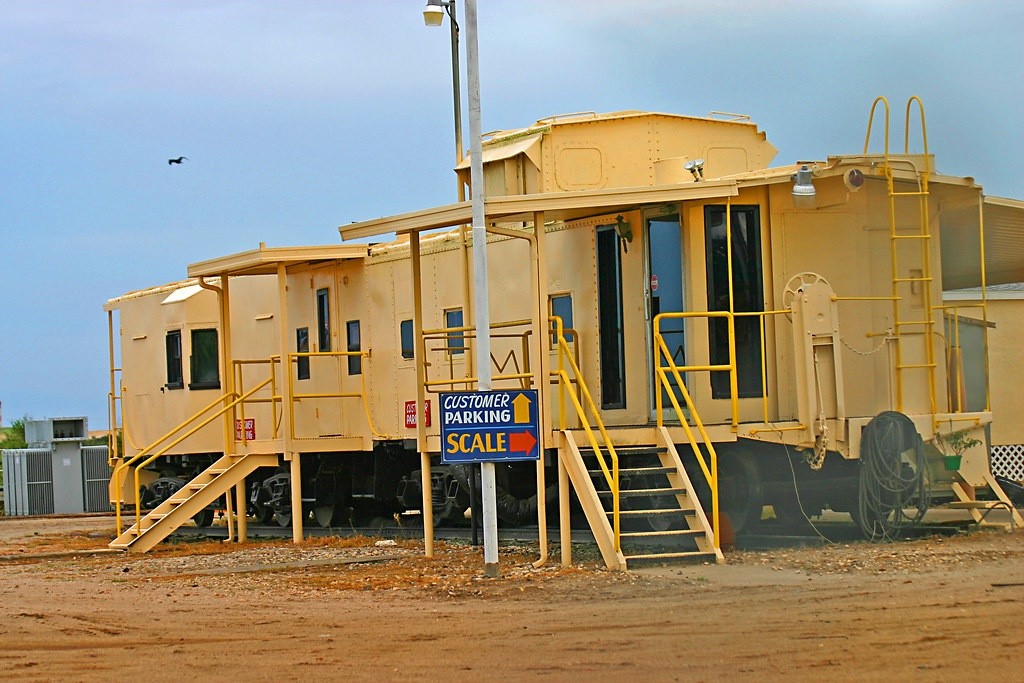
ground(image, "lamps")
xmin=791 ymin=166 xmax=816 ymax=208
xmin=614 ymin=214 xmax=631 ymax=253
xmin=685 ymin=160 xmax=705 ymax=182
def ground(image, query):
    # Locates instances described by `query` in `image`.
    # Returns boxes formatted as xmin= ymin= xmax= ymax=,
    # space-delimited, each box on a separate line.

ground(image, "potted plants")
xmin=934 ymin=431 xmax=982 ymax=470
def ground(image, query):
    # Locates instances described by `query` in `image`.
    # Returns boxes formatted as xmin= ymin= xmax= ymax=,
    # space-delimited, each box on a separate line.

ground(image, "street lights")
xmin=420 ymin=0 xmax=479 ymax=547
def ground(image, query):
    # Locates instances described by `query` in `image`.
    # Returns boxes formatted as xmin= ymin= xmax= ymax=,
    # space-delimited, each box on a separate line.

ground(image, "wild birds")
xmin=168 ymin=156 xmax=190 ymax=165
